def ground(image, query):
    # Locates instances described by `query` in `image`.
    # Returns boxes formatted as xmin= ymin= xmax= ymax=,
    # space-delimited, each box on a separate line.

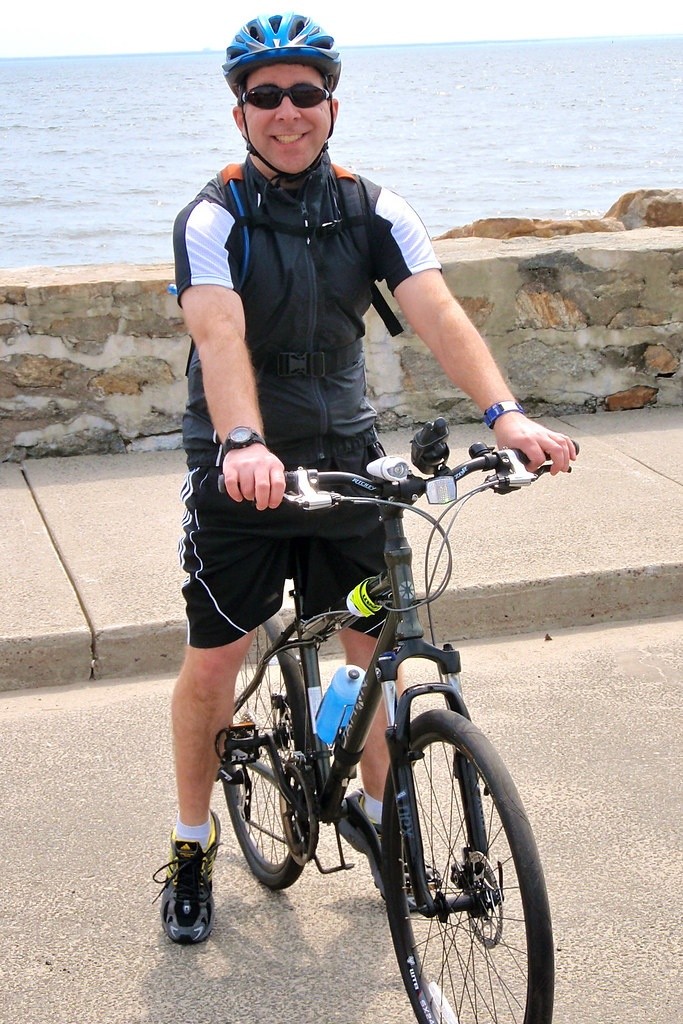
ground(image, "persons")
xmin=150 ymin=12 xmax=578 ymax=946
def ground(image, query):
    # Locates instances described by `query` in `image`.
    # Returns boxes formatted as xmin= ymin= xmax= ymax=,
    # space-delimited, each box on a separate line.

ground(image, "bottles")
xmin=315 ymin=664 xmax=367 ymax=744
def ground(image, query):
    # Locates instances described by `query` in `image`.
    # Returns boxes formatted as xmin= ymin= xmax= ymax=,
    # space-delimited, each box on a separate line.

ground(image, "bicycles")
xmin=211 ymin=425 xmax=579 ymax=1024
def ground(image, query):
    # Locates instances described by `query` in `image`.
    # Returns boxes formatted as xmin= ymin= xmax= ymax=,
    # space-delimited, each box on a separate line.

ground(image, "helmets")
xmin=222 ymin=12 xmax=341 ymax=99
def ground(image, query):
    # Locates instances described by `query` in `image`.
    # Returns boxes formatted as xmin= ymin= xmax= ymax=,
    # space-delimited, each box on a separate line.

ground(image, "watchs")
xmin=219 ymin=424 xmax=267 ymax=455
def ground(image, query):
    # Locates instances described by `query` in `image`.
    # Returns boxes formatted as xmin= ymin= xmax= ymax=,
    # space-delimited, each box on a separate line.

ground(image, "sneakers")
xmin=339 ymin=788 xmax=435 ymax=908
xmin=151 ymin=807 xmax=224 ymax=944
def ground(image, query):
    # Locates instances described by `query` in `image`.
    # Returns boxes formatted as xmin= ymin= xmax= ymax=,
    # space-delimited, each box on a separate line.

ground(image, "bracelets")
xmin=483 ymin=401 xmax=527 ymax=430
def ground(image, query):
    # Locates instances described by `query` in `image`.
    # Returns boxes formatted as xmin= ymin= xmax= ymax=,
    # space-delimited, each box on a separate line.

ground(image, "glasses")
xmin=243 ymin=83 xmax=330 ymax=110
